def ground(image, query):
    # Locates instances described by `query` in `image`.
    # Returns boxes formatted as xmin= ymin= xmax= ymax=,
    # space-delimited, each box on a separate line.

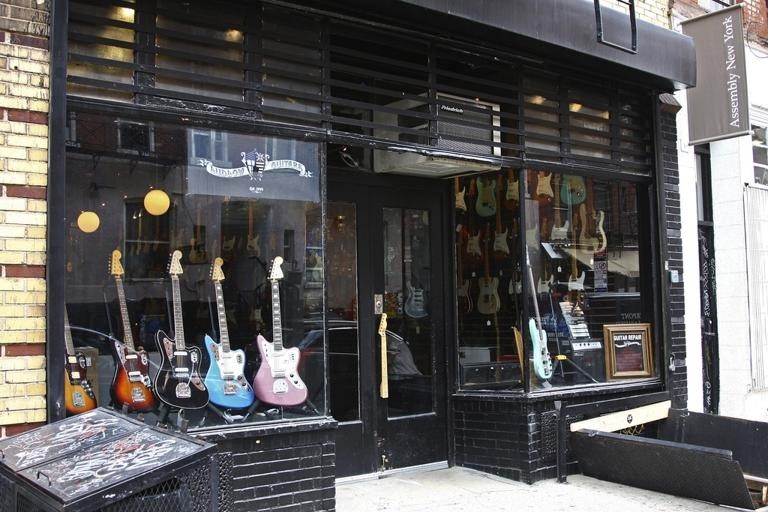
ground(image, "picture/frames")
xmin=604 ymin=322 xmax=654 ymax=383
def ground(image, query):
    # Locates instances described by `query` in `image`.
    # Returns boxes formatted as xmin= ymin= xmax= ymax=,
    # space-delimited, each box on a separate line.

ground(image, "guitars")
xmin=526 ymin=261 xmax=555 ymax=378
xmin=61 ymin=300 xmax=98 ymax=412
xmin=204 ymin=255 xmax=253 ymax=409
xmin=189 ymin=196 xmax=260 ymax=264
xmin=254 ymin=255 xmax=308 ymax=407
xmin=404 ymin=277 xmax=429 ymax=319
xmin=154 ymin=249 xmax=208 ymax=409
xmin=456 ymin=170 xmax=609 ymax=316
xmin=106 ymin=251 xmax=160 ymax=413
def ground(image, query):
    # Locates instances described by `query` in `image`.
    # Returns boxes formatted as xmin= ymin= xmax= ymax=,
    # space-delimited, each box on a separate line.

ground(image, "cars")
xmin=67 ymin=325 xmax=197 ymax=403
xmin=241 ymin=315 xmax=423 ymax=385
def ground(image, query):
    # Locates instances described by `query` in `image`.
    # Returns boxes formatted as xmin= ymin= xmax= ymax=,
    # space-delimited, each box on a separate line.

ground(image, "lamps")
xmin=145 ymin=118 xmax=171 ymax=218
xmin=77 ymin=210 xmax=101 ymax=233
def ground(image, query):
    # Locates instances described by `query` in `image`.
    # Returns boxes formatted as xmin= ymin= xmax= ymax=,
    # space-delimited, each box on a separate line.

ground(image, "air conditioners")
xmin=375 ymin=93 xmax=501 ymax=182
